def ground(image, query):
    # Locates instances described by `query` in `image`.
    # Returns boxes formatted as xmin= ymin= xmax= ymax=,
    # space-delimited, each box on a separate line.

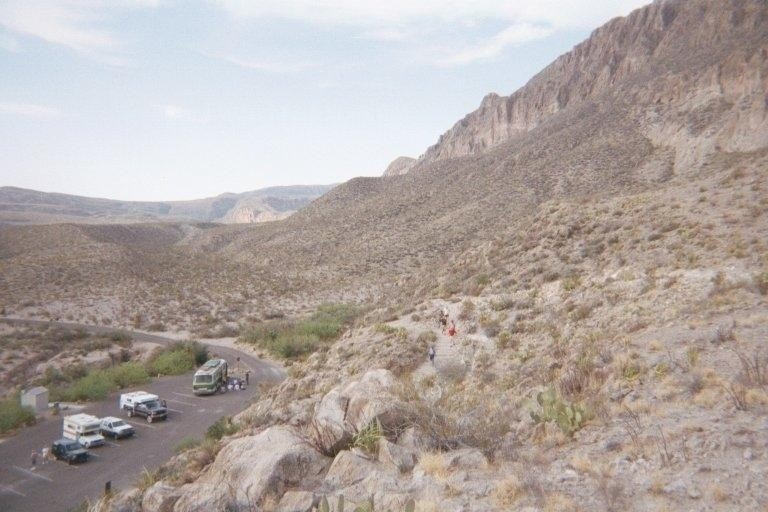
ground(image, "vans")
xmin=61 ymin=414 xmax=105 ymax=447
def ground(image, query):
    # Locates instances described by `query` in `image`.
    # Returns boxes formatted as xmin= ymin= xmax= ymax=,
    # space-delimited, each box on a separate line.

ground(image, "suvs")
xmin=52 ymin=439 xmax=89 ymax=463
xmin=101 ymin=416 xmax=133 ymax=439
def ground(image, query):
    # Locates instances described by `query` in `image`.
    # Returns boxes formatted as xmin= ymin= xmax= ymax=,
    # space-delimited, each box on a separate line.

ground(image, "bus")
xmin=193 ymin=359 xmax=228 ymax=395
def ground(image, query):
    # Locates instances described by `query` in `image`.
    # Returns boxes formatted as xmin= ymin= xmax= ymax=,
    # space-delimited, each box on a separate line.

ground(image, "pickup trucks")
xmin=120 ymin=391 xmax=167 ymax=422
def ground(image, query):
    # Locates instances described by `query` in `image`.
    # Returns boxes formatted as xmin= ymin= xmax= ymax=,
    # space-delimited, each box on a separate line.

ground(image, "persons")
xmin=447 ymin=320 xmax=456 ymax=346
xmin=30 ymin=447 xmax=38 ymax=472
xmin=428 ymin=345 xmax=437 ymax=364
xmin=222 ymin=373 xmax=227 ymax=385
xmin=42 ymin=443 xmax=50 ymax=464
xmin=440 ymin=316 xmax=449 ymax=333
xmin=245 ymin=371 xmax=249 ymax=385
